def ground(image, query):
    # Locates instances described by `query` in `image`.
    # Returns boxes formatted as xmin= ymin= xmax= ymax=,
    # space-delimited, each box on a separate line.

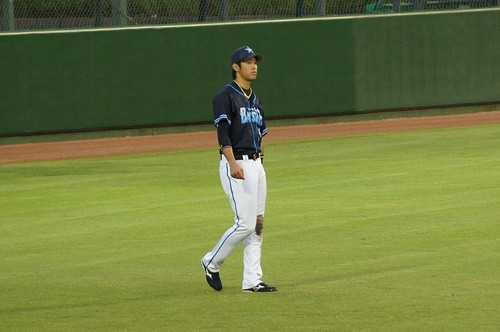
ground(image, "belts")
xmin=234 ymin=153 xmax=260 ymax=160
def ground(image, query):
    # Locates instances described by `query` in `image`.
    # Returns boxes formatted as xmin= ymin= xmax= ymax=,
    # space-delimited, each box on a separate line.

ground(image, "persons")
xmin=200 ymin=46 xmax=278 ymax=293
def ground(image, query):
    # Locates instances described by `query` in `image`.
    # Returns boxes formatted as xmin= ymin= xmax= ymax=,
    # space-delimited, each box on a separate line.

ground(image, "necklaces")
xmin=233 ymin=79 xmax=253 ymax=99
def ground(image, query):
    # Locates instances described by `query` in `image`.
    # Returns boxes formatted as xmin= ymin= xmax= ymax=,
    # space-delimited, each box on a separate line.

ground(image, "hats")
xmin=230 ymin=45 xmax=264 ymax=63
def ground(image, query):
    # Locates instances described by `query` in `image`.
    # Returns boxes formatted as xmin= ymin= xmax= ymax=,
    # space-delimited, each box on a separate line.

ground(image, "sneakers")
xmin=241 ymin=282 xmax=278 ymax=293
xmin=200 ymin=258 xmax=223 ymax=292
